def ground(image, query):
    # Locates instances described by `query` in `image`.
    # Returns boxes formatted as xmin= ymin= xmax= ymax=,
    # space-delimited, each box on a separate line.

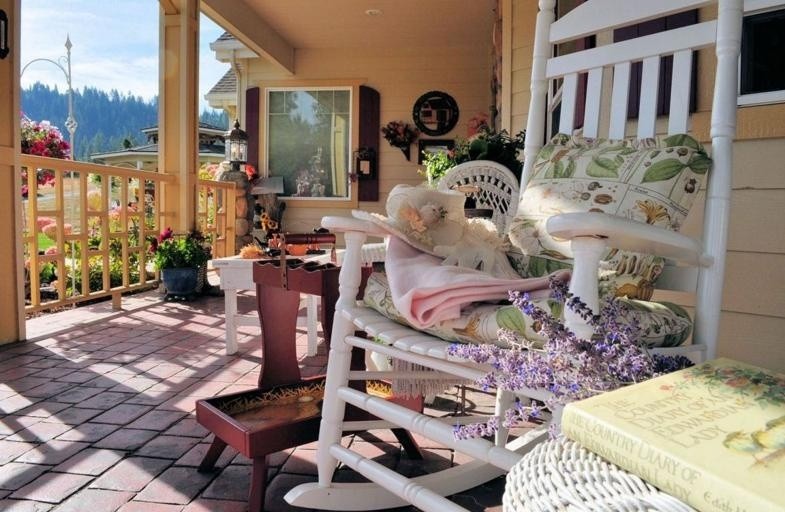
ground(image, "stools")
xmin=501 ymin=433 xmax=700 ymax=512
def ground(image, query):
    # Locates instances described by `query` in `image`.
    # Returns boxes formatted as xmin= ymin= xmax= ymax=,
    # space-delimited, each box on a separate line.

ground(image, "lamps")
xmin=222 ymin=118 xmax=249 ymax=171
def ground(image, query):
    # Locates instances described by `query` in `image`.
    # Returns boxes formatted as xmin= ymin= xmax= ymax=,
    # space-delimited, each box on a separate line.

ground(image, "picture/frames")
xmin=418 ymin=139 xmax=454 ymax=164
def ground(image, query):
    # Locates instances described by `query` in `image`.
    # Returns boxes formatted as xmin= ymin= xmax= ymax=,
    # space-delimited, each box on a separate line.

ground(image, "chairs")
xmin=359 ymin=160 xmax=519 ymax=262
xmin=283 ymin=1 xmax=744 ymax=512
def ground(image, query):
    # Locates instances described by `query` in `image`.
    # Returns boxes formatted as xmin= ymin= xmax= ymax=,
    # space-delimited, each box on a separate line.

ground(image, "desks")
xmin=207 ymin=249 xmax=346 ymax=357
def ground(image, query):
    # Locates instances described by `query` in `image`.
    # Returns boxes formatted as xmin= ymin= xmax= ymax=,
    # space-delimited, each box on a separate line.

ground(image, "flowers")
xmin=145 ymin=226 xmax=210 ymax=271
xmin=20 ymin=111 xmax=71 ymax=200
xmin=381 ymin=120 xmax=420 ymax=147
xmin=467 ymin=114 xmax=490 ymax=136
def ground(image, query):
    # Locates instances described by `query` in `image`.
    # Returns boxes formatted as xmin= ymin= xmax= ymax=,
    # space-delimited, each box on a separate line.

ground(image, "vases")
xmin=159 ymin=267 xmax=198 ymax=303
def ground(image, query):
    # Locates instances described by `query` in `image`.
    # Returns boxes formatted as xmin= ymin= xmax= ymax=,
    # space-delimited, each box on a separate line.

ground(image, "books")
xmin=562 ymin=357 xmax=785 ymax=511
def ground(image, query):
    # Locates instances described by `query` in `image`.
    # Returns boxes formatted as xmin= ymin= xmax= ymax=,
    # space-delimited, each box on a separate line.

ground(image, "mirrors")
xmin=413 ymin=91 xmax=459 ymax=136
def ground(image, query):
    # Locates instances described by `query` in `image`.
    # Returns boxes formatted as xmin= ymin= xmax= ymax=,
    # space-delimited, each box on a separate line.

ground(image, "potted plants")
xmin=417 ymin=148 xmax=455 ymax=185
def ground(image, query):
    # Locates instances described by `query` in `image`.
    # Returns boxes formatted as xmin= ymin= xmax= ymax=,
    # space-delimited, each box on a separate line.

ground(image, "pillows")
xmin=508 ymin=133 xmax=713 ymax=283
